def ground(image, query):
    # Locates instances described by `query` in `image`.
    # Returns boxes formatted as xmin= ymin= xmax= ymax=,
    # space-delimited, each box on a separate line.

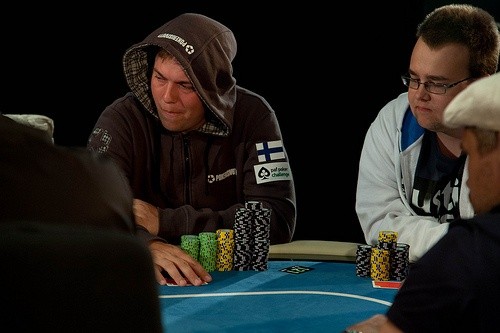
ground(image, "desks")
xmin=154 ymin=240 xmax=419 ymax=333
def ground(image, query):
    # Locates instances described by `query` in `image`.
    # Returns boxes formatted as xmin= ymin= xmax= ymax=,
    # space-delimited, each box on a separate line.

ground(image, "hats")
xmin=442 ymin=72 xmax=500 ymax=131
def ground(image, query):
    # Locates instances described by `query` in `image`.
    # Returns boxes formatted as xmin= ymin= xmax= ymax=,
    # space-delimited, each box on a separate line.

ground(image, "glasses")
xmin=401 ymin=73 xmax=472 ymax=95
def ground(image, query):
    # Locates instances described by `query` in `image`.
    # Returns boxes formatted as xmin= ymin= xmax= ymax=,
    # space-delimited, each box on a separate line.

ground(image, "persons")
xmin=354 ymin=3 xmax=500 ymax=262
xmin=88 ymin=13 xmax=295 ymax=286
xmin=344 ymin=72 xmax=500 ymax=333
xmin=0 ymin=111 xmax=167 ymax=333
